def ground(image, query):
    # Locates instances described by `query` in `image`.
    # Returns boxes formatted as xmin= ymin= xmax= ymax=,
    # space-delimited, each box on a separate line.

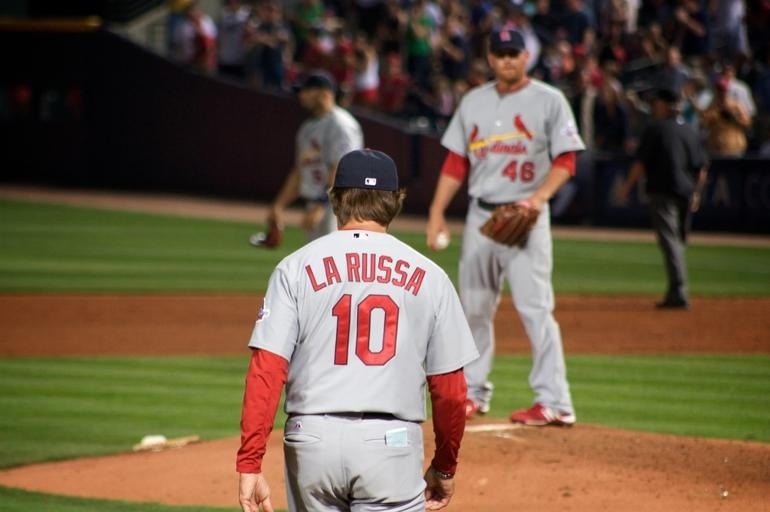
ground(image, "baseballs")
xmin=436 ymin=231 xmax=449 ymax=250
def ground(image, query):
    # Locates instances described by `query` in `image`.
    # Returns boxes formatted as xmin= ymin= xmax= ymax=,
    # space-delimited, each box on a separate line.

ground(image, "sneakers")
xmin=511 ymin=403 xmax=577 ymax=425
xmin=656 ymin=299 xmax=688 ymax=308
xmin=465 ymin=400 xmax=489 ymax=418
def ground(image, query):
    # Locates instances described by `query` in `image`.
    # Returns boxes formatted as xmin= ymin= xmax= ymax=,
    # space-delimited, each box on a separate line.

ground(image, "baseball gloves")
xmin=480 ymin=203 xmax=539 ymax=248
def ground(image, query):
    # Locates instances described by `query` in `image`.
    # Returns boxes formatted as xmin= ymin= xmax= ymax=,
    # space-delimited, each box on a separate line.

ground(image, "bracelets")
xmin=430 ymin=464 xmax=455 ymax=480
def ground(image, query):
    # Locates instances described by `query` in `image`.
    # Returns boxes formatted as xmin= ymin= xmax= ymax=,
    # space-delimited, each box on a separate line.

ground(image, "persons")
xmin=261 ymin=69 xmax=365 ymax=251
xmin=618 ymin=87 xmax=712 ymax=309
xmin=237 ymin=147 xmax=481 ymax=512
xmin=426 ymin=26 xmax=576 ymax=426
xmin=169 ymin=0 xmax=770 ymax=158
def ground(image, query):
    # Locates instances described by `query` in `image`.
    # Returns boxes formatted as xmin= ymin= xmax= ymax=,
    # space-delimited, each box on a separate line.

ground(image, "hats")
xmin=490 ymin=30 xmax=525 ymax=54
xmin=334 ymin=149 xmax=398 ymax=190
xmin=292 ymin=73 xmax=332 ymax=90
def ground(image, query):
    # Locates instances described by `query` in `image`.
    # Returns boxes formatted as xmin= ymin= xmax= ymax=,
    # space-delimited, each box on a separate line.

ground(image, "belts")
xmin=330 ymin=410 xmax=399 ymax=419
xmin=468 ymin=197 xmax=513 ymax=211
